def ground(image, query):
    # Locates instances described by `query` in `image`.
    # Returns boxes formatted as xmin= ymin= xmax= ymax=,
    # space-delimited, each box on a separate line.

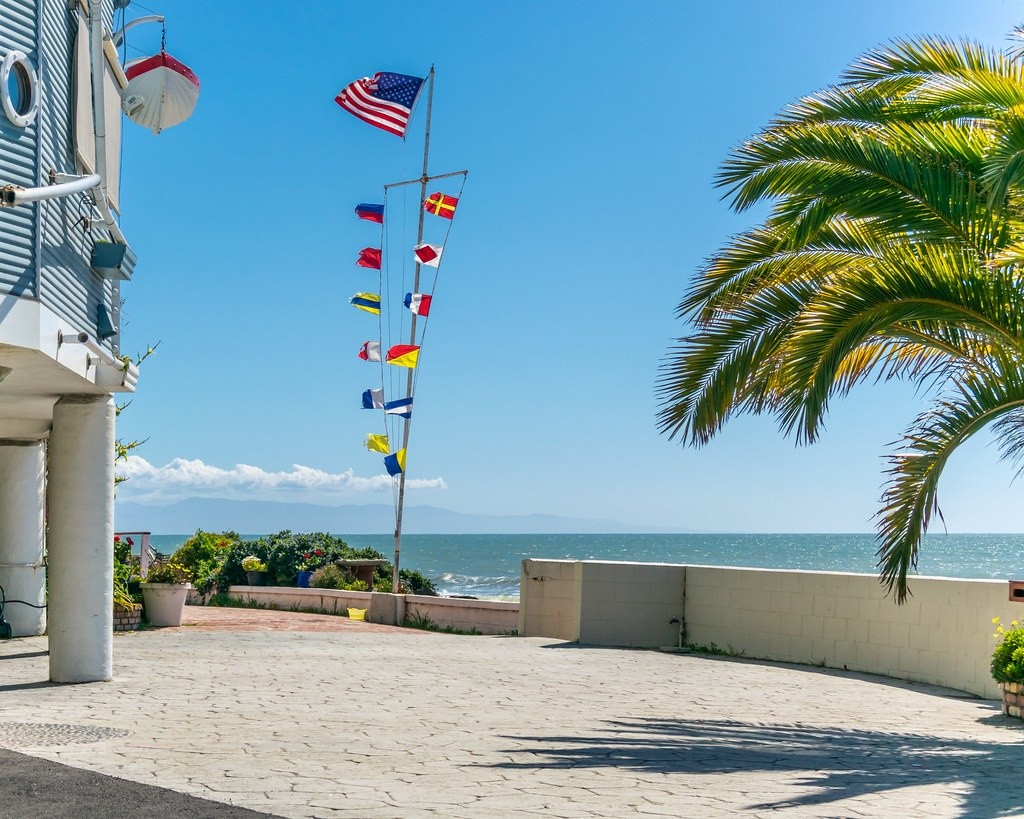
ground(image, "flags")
xmin=349 ymin=291 xmax=382 ymax=317
xmin=402 ymin=289 xmax=430 ymax=316
xmin=386 ymin=341 xmax=420 ymax=372
xmin=332 ymin=72 xmax=424 ymax=138
xmin=384 ymin=449 xmax=409 ymax=478
xmin=382 ymin=396 xmax=415 ymax=419
xmin=360 ymin=388 xmax=386 ymax=409
xmin=362 ymin=433 xmax=390 ymax=455
xmin=358 ymin=341 xmax=382 ymax=363
xmin=422 ymin=190 xmax=460 ymax=222
xmin=354 ymin=202 xmax=384 ymax=224
xmin=413 ymin=240 xmax=444 ymax=269
xmin=357 ymin=246 xmax=382 ymax=271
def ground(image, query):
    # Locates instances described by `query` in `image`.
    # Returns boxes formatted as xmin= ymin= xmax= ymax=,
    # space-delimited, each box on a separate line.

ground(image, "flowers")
xmin=295 ymin=550 xmax=325 ymax=572
xmin=146 ymin=564 xmax=196 ymax=585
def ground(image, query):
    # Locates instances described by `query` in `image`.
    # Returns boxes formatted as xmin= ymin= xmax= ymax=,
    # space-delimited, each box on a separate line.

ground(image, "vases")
xmin=140 ymin=581 xmax=192 ymax=627
xmin=298 ymin=572 xmax=314 ymax=587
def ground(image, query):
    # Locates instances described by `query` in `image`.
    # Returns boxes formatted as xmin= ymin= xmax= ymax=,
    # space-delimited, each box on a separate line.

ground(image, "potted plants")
xmin=241 ymin=555 xmax=269 ymax=587
